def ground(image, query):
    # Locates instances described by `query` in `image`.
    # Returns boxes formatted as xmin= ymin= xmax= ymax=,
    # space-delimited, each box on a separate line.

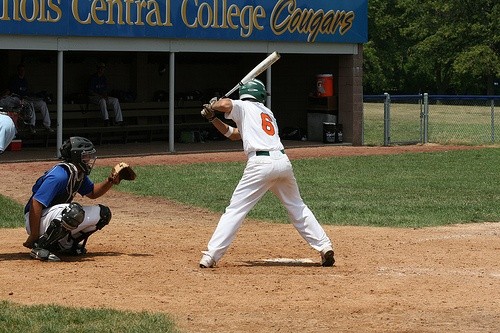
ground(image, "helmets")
xmin=239 ymin=79 xmax=271 ymax=103
xmin=0 ymin=93 xmax=34 ymax=131
xmin=58 ymin=137 xmax=97 ymax=175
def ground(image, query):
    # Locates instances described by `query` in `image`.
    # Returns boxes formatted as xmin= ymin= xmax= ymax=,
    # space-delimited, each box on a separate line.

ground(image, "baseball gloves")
xmin=108 ymin=161 xmax=137 ymax=186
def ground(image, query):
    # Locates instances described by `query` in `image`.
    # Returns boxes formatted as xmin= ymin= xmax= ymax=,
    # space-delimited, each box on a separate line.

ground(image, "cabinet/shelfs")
xmin=306 ymin=96 xmax=339 ymax=141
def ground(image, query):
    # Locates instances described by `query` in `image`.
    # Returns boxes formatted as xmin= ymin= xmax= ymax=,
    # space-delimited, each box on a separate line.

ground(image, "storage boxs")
xmin=284 ymin=127 xmax=304 ymax=141
xmin=7 ymin=140 xmax=26 ymax=160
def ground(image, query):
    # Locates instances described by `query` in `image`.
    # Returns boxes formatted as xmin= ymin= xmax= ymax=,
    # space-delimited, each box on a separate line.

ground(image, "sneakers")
xmin=55 ymin=244 xmax=87 ymax=254
xmin=29 ymin=247 xmax=60 ymax=262
xmin=319 ymin=247 xmax=336 ymax=267
xmin=200 ymin=254 xmax=216 ymax=268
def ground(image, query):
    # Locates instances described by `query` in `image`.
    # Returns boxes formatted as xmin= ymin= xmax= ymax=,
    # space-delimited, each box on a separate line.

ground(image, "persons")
xmin=7 ymin=64 xmax=54 ymax=134
xmin=87 ymin=62 xmax=126 ymax=126
xmin=0 ymin=96 xmax=36 ymax=155
xmin=23 ymin=137 xmax=136 ymax=261
xmin=200 ymin=79 xmax=335 ymax=268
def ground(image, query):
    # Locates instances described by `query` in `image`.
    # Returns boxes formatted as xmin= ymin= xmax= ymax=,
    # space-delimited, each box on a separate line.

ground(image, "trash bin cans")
xmin=304 ymin=95 xmax=337 ymax=141
xmin=323 ymin=122 xmax=338 ymax=143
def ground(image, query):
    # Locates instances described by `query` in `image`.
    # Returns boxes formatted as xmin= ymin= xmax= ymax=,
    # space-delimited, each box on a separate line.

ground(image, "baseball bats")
xmin=201 ymin=50 xmax=281 ymax=118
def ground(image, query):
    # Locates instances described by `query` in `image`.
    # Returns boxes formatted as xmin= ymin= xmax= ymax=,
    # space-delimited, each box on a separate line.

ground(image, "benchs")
xmin=18 ymin=101 xmax=222 ymax=148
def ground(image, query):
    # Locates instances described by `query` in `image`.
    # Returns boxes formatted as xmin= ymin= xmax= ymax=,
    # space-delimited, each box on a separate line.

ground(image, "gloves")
xmin=200 ymin=104 xmax=216 ymax=122
xmin=210 ymin=95 xmax=226 ymax=103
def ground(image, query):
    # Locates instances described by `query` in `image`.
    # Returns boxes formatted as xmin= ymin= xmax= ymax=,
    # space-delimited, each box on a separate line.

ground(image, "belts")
xmin=256 ymin=150 xmax=287 ymax=156
